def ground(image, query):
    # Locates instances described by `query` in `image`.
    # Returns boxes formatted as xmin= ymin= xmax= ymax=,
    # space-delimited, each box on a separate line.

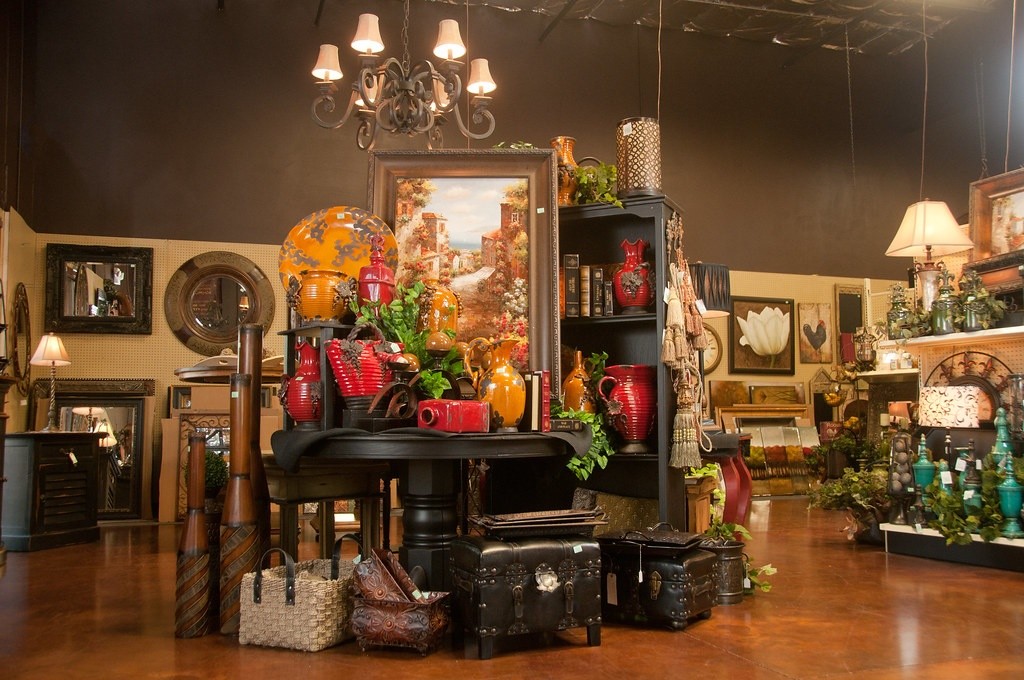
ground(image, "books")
xmin=550 ymin=420 xmax=582 ymax=432
xmin=604 ymin=281 xmax=613 ymax=317
xmin=579 ymin=265 xmax=591 ymax=317
xmin=518 ymin=370 xmax=551 ymax=433
xmin=559 ymin=254 xmax=579 ymax=319
xmin=592 ymin=268 xmax=604 ymax=316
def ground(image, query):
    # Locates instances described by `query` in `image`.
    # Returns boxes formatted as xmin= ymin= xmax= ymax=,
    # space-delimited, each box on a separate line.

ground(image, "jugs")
xmin=463 ymin=337 xmax=526 ymax=433
xmin=285 ymin=269 xmax=357 ymax=326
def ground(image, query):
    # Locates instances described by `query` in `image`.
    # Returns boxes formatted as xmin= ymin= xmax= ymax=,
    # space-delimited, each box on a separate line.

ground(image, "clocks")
xmin=694 ymin=321 xmax=723 ymax=375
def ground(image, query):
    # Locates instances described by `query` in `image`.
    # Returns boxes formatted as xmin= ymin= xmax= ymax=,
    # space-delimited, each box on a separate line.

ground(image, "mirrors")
xmin=30 ymin=376 xmax=156 ymax=527
xmin=11 ymin=282 xmax=32 ymax=397
xmin=175 ymin=413 xmax=230 ymax=522
xmin=163 ymin=250 xmax=275 ymax=357
xmin=44 ymin=243 xmax=154 ymax=335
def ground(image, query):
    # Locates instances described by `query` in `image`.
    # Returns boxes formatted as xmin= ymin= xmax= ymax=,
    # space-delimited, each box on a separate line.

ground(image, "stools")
xmin=444 ymin=538 xmax=602 ymax=660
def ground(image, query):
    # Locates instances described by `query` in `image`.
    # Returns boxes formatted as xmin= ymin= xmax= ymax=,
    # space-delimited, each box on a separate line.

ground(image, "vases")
xmin=612 ymin=237 xmax=656 ymax=315
xmin=550 ymin=135 xmax=578 ymax=206
xmin=278 ymin=340 xmax=322 ymax=431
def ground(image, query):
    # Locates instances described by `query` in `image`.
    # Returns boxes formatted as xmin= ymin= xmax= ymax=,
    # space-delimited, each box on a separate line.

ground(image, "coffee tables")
xmin=277 ymin=322 xmax=354 ymax=432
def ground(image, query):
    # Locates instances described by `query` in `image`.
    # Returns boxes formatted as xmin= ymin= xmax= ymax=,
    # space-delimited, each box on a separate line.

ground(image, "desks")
xmin=698 ymin=433 xmax=754 ymax=544
xmin=303 ymin=433 xmax=569 ymax=592
xmin=260 ymin=447 xmax=384 ymax=566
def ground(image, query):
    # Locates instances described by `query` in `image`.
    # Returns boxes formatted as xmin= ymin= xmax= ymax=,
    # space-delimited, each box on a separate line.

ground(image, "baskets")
xmin=238 ymin=535 xmax=365 ymax=651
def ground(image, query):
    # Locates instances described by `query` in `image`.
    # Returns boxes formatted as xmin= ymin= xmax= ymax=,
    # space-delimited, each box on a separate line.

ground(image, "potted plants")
xmin=804 ymin=469 xmax=890 ymax=547
xmin=689 ymin=463 xmax=777 ymax=603
xmin=183 ymin=448 xmax=228 ymax=544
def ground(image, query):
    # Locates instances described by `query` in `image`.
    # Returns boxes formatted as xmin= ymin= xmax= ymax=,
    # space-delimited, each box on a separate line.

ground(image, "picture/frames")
xmin=727 ymin=296 xmax=795 ymax=377
xmin=966 ymin=166 xmax=1024 ymax=262
xmin=714 ymin=404 xmax=810 ymax=431
xmin=170 ymin=384 xmax=272 ymax=410
xmin=365 ymin=147 xmax=561 ymax=399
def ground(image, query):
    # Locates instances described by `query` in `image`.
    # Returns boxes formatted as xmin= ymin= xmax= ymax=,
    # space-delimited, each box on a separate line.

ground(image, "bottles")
xmin=598 ymin=363 xmax=658 ymax=454
xmin=279 ymin=341 xmax=324 ymax=423
xmin=612 ymin=239 xmax=657 ymax=315
xmin=418 ymin=398 xmax=491 ymax=434
xmin=560 ymin=350 xmax=596 ymax=416
xmin=547 ymin=135 xmax=580 ymax=207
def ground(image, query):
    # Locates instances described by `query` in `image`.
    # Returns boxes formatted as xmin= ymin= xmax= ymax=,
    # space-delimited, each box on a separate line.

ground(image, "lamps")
xmin=885 ymin=197 xmax=974 ymax=314
xmin=310 ymin=0 xmax=498 ymax=151
xmin=94 ymin=420 xmax=117 ymax=447
xmin=71 ymin=406 xmax=104 ymax=432
xmin=617 ymin=117 xmax=662 ymax=199
xmin=29 ymin=331 xmax=71 ymax=431
xmin=917 ymin=386 xmax=980 ymax=466
xmin=688 ymin=261 xmax=730 ymax=430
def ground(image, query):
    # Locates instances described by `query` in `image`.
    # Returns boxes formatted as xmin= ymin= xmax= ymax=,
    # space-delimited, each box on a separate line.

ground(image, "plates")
xmin=277 ymin=205 xmax=398 ymax=302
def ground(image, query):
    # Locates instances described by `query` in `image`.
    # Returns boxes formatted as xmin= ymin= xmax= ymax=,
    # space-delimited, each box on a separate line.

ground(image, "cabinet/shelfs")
xmin=560 ymin=196 xmax=687 ymax=537
xmin=99 ymin=450 xmax=122 ymax=510
xmin=1 ymin=432 xmax=109 ymax=552
